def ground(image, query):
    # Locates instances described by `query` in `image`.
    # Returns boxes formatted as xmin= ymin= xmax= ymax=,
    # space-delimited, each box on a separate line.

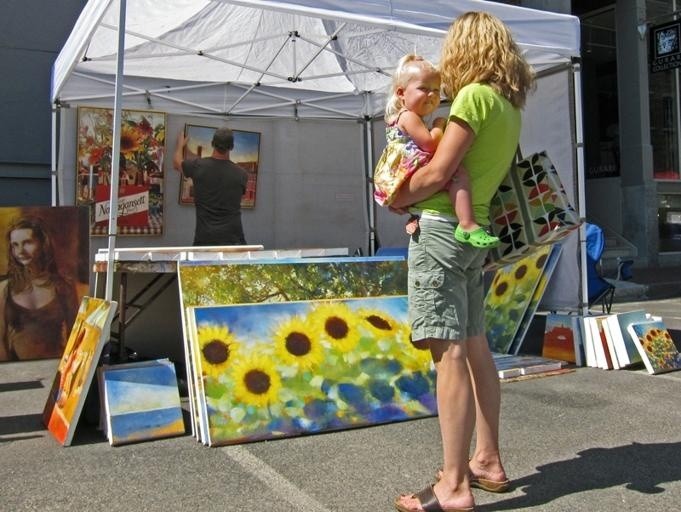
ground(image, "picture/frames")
xmin=177 ymin=123 xmax=261 ymax=209
xmin=75 ymin=106 xmax=165 ymax=235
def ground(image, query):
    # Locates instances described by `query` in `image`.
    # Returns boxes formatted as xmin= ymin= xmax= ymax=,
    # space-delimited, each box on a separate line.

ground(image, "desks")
xmin=91 ymin=259 xmax=177 ymax=363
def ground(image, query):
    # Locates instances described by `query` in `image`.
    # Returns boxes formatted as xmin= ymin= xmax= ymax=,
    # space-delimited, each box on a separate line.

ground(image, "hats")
xmin=213 ymin=128 xmax=233 ymax=150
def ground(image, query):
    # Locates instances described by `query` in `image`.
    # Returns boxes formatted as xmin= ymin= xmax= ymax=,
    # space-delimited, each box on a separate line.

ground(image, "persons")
xmin=369 ymin=52 xmax=501 ymax=252
xmin=0 ymin=215 xmax=81 ymax=364
xmin=393 ymin=9 xmax=543 ymax=510
xmin=173 ymin=126 xmax=252 ymax=248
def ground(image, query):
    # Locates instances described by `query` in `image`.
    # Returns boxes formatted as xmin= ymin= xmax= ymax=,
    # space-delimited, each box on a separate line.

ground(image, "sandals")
xmin=455 ymin=223 xmax=501 ymax=249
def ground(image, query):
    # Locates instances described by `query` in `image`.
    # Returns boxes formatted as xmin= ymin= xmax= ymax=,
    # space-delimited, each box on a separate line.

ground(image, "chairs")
xmin=586 ymin=222 xmax=616 ymax=314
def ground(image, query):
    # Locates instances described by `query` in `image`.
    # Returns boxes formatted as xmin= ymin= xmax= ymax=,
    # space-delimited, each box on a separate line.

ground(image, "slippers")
xmin=433 ymin=457 xmax=510 ymax=493
xmin=394 ymin=484 xmax=475 ymax=512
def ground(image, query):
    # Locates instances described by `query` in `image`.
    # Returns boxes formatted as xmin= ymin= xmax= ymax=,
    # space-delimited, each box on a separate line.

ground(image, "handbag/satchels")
xmin=483 ymin=152 xmax=579 ymax=272
